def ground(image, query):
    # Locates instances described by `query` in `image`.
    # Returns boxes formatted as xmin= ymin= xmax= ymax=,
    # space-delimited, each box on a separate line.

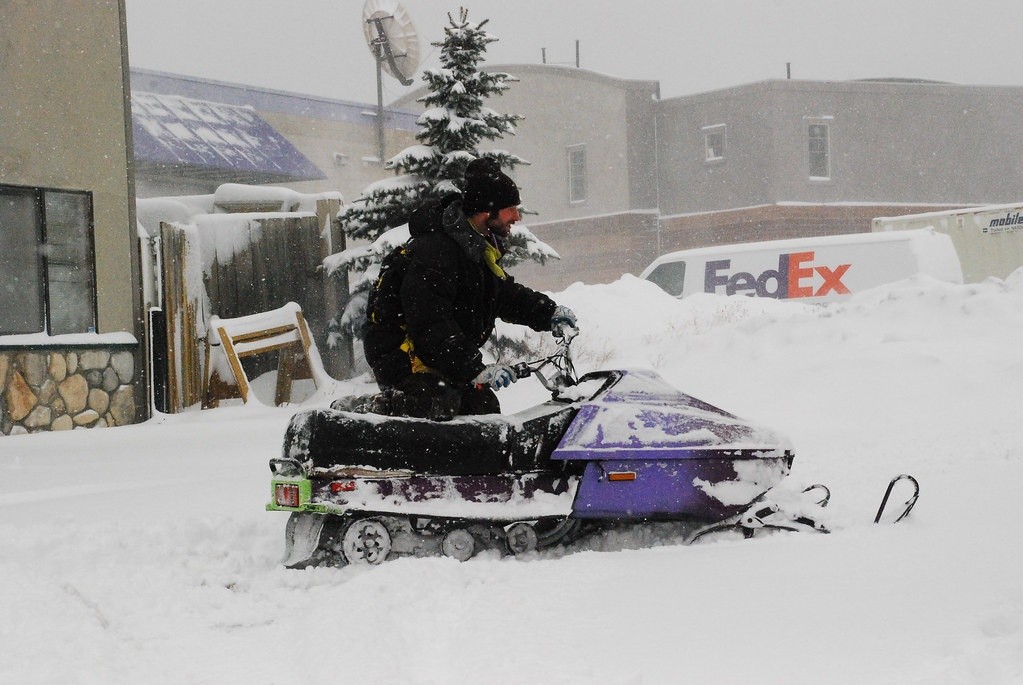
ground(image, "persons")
xmin=359 ymin=158 xmax=579 ymax=421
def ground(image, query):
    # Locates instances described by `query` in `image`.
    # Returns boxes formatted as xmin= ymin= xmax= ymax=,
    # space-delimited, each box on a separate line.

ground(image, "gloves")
xmin=471 ymin=363 xmax=519 ymax=392
xmin=549 ymin=306 xmax=576 ymax=338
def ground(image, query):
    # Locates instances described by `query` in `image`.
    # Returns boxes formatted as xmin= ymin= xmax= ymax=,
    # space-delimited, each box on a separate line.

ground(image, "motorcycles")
xmin=265 ymin=324 xmax=830 ymax=569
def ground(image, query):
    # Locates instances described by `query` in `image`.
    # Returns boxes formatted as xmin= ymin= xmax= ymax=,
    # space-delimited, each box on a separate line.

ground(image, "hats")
xmin=463 ymin=157 xmax=520 ymax=212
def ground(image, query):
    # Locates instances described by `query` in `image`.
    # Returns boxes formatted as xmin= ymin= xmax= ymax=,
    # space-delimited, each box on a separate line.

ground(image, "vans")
xmin=636 ymin=227 xmax=967 ymax=303
